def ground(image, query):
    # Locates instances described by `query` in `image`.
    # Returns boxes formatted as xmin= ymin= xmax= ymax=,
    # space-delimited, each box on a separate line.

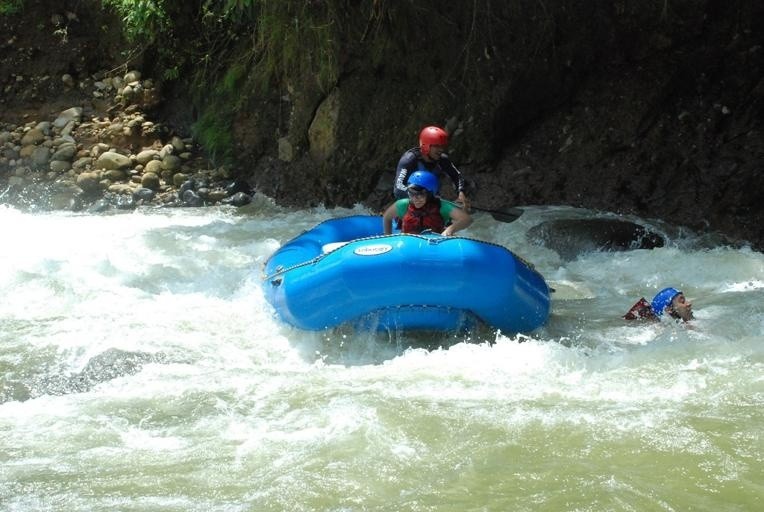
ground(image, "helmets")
xmin=419 ymin=126 xmax=450 ymax=159
xmin=650 ymin=288 xmax=683 ymax=326
xmin=407 ymin=170 xmax=440 ymax=195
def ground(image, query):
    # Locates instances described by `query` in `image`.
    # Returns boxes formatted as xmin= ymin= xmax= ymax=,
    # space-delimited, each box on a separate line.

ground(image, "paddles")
xmin=419 ymin=228 xmax=577 ymax=299
xmin=458 ymin=202 xmax=524 ymax=222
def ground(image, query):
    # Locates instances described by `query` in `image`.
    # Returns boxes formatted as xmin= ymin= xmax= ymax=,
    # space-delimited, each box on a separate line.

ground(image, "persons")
xmin=393 ymin=126 xmax=470 ymax=210
xmin=382 ymin=171 xmax=473 ymax=236
xmin=650 ymin=287 xmax=703 ymax=332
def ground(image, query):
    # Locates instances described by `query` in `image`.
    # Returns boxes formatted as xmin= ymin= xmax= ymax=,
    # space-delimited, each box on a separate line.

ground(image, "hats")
xmin=405 ymin=185 xmax=424 ymax=192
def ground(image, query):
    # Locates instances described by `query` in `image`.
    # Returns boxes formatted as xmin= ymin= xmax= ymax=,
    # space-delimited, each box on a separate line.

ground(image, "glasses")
xmin=407 ymin=190 xmax=427 ymax=198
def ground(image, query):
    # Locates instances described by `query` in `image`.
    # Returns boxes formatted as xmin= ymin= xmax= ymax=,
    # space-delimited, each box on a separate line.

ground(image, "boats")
xmin=258 ymin=207 xmax=555 ymax=343
